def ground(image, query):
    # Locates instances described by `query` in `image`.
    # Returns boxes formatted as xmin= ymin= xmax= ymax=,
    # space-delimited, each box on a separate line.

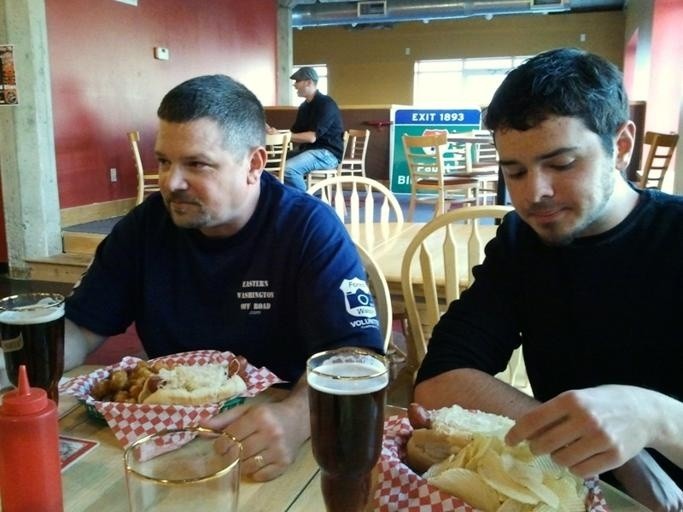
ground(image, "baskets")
xmin=75 ymin=354 xmax=246 ymax=421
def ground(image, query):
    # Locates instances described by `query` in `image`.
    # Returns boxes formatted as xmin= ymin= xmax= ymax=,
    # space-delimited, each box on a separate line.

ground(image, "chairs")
xmin=400 ymin=205 xmax=537 ymax=399
xmin=351 ymin=239 xmax=393 ymax=354
xmin=128 ymin=131 xmax=161 ymax=206
xmin=634 ymin=129 xmax=678 ymax=188
xmin=263 ymin=128 xmax=373 ymax=184
xmin=308 ymin=176 xmax=405 ymax=223
xmin=401 ymin=121 xmax=500 ymax=222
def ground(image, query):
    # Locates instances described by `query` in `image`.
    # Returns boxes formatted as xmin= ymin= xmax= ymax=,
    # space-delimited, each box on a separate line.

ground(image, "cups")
xmin=305 ymin=346 xmax=390 ymax=511
xmin=121 ymin=426 xmax=241 ymax=510
xmin=0 ymin=289 xmax=67 ymax=409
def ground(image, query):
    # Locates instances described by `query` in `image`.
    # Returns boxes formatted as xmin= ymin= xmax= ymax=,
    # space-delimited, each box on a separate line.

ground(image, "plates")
xmin=73 ymin=380 xmax=247 ymax=426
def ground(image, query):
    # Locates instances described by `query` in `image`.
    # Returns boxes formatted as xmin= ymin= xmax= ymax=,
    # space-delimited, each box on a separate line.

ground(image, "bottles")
xmin=0 ymin=364 xmax=65 ymax=512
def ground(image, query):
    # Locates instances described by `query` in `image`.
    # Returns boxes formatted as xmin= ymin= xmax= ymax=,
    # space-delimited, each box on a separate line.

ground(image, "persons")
xmin=49 ymin=75 xmax=389 ymax=489
xmin=264 ymin=64 xmax=345 ymax=193
xmin=408 ymin=44 xmax=683 ymax=484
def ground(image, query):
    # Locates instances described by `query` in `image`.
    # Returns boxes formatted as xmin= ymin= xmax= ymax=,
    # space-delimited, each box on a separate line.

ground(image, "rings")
xmin=252 ymin=451 xmax=267 ymax=468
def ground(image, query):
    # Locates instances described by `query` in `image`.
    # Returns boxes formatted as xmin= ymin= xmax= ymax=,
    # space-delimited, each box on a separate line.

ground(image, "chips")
xmin=421 ymin=434 xmax=589 ymax=512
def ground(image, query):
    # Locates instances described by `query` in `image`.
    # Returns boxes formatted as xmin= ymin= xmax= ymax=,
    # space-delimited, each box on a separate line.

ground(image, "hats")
xmin=290 ymin=67 xmax=318 ymax=80
